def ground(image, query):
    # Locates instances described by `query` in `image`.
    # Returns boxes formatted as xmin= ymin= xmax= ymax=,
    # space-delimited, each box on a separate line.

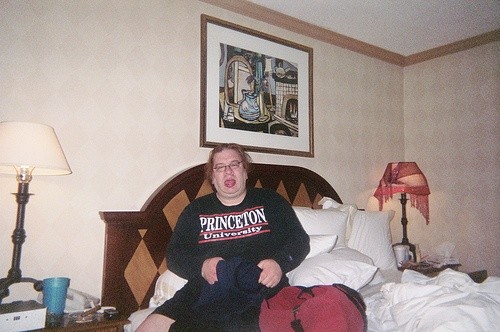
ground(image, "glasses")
xmin=213 ymin=161 xmax=243 ymax=172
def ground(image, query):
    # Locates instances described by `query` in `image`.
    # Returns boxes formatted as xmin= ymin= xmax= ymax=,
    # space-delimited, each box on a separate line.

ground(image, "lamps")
xmin=0 ymin=121 xmax=73 ymax=304
xmin=373 ymin=162 xmax=430 ymax=262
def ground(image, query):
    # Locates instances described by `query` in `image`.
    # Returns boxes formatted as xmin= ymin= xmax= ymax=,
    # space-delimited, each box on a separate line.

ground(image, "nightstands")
xmin=398 ymin=264 xmax=461 ymax=278
xmin=25 ymin=313 xmax=130 ymax=332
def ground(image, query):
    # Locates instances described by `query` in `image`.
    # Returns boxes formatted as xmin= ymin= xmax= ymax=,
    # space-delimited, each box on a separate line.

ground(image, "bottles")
xmin=415 ymin=244 xmax=421 ymax=263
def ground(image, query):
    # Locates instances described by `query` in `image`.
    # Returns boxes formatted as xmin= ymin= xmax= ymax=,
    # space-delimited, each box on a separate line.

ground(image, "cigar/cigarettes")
xmin=81 ymin=305 xmax=101 ymax=318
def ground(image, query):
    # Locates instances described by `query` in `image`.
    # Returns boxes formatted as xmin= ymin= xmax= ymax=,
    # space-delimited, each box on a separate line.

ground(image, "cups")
xmin=43 ymin=277 xmax=70 ymax=317
xmin=393 ymin=244 xmax=414 ymax=268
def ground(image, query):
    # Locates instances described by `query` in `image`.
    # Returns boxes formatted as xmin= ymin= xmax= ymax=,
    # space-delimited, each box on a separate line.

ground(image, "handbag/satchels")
xmin=257 ymin=283 xmax=367 ymax=332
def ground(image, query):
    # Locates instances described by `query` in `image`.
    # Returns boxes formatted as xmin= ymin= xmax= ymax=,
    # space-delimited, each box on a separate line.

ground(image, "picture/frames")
xmin=201 ymin=14 xmax=314 ymax=159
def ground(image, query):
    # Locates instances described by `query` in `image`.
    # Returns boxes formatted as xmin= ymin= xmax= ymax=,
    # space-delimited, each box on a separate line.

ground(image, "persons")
xmin=133 ymin=143 xmax=310 ymax=332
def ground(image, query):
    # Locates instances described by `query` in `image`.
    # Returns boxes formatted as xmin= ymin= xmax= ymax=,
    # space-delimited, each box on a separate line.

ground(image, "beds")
xmin=99 ymin=163 xmax=500 ymax=332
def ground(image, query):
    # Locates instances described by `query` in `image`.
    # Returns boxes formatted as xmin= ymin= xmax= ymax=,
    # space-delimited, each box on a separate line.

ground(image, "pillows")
xmin=149 ymin=197 xmax=397 ymax=308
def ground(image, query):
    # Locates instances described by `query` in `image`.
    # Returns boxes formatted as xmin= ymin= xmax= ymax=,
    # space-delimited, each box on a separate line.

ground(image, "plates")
xmin=68 ymin=310 xmax=98 ymax=323
